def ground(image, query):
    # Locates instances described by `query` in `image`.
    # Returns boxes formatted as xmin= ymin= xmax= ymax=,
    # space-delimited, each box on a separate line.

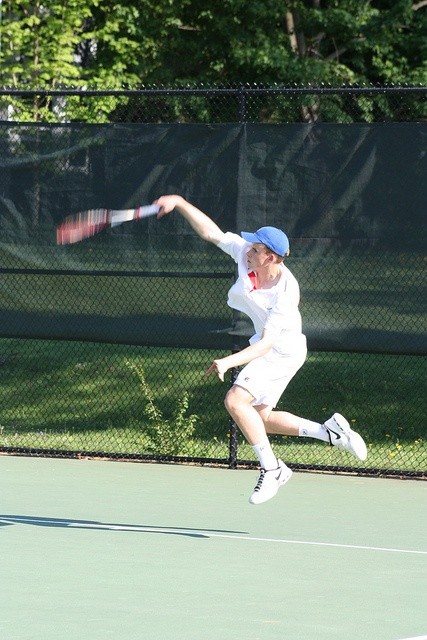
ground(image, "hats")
xmin=241 ymin=226 xmax=290 ymax=257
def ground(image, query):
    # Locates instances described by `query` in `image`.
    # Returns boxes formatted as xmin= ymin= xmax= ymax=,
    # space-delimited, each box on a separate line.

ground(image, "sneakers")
xmin=249 ymin=458 xmax=293 ymax=504
xmin=322 ymin=412 xmax=367 ymax=461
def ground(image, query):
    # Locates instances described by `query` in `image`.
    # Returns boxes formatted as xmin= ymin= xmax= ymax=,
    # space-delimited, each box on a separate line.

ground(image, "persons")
xmin=152 ymin=193 xmax=367 ymax=506
